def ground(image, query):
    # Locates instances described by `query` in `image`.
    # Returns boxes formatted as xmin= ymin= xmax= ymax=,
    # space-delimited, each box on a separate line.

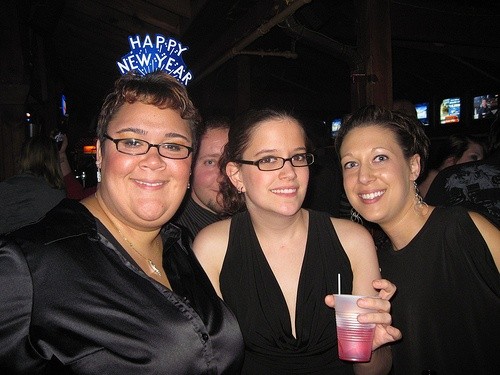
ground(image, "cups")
xmin=333 ymin=294 xmax=382 ymax=362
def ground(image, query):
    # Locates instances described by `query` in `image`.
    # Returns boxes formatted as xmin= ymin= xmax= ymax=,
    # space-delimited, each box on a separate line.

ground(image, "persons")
xmin=192 ymin=109 xmax=403 ymax=375
xmin=0 ymin=71 xmax=245 ymax=375
xmin=418 ymin=126 xmax=500 ymax=228
xmin=336 ymin=106 xmax=499 ymax=375
xmin=171 ymin=118 xmax=245 ymax=237
xmin=478 ymin=99 xmax=494 ymax=119
xmin=0 ymin=108 xmax=100 ymax=234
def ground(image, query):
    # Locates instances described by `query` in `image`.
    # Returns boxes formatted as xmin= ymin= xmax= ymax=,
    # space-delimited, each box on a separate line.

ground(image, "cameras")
xmin=53 ymin=129 xmax=64 ymax=151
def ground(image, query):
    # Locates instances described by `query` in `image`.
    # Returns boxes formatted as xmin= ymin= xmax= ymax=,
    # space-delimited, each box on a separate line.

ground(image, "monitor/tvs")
xmin=331 ymin=115 xmax=342 ymax=137
xmin=413 ymin=91 xmax=500 ymax=126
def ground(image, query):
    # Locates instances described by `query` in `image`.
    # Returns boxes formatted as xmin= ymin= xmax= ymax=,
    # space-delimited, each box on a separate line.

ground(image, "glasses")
xmin=103 ymin=134 xmax=194 ymax=160
xmin=237 ymin=152 xmax=315 ymax=171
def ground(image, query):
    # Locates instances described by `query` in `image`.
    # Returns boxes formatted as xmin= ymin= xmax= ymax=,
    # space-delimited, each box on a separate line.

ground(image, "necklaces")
xmin=118 ymin=229 xmax=163 ymax=277
